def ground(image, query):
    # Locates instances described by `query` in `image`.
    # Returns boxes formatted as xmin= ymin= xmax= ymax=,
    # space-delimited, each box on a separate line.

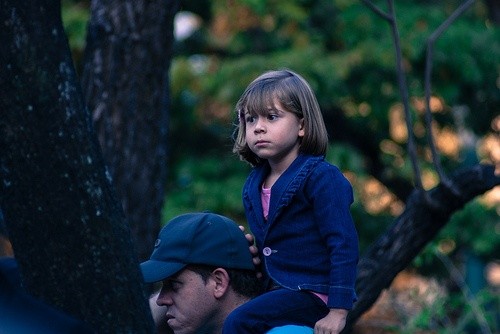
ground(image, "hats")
xmin=140 ymin=212 xmax=254 ymax=283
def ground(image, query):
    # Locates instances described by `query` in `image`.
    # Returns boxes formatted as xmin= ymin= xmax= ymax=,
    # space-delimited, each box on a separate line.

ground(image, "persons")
xmin=131 ymin=210 xmax=314 ymax=334
xmin=221 ymin=70 xmax=361 ymax=334
